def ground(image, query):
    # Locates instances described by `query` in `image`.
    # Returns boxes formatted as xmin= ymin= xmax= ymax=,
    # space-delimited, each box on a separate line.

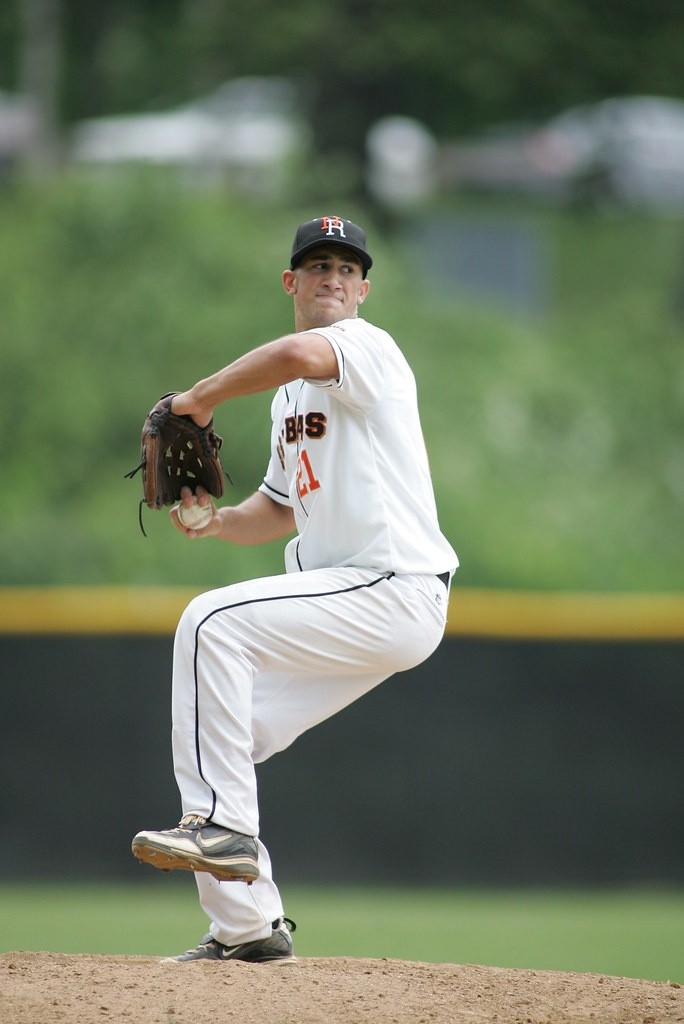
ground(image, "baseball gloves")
xmin=140 ymin=391 xmax=229 ymax=509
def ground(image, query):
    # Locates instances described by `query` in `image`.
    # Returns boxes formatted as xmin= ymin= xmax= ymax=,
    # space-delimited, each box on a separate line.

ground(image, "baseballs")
xmin=172 ymin=496 xmax=215 ymax=531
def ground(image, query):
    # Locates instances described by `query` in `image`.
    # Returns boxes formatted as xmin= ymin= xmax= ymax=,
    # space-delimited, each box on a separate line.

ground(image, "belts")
xmin=435 ymin=572 xmax=449 ymax=589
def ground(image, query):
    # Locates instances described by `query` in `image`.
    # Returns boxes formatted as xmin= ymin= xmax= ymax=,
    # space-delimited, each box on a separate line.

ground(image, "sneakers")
xmin=160 ymin=917 xmax=297 ymax=966
xmin=132 ymin=815 xmax=260 ymax=886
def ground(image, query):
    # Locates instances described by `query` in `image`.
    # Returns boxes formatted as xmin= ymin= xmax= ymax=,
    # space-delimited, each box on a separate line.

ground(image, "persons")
xmin=132 ymin=216 xmax=460 ymax=966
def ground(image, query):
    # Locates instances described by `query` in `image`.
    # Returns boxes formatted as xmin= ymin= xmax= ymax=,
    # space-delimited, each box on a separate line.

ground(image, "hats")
xmin=290 ymin=216 xmax=372 ymax=280
